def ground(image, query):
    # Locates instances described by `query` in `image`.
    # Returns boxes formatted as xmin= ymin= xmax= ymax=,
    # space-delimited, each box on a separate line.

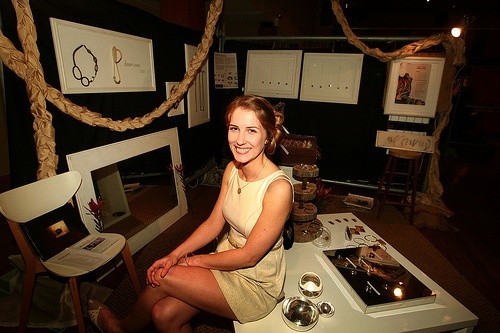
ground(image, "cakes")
xmin=285 ymin=163 xmax=323 ymax=243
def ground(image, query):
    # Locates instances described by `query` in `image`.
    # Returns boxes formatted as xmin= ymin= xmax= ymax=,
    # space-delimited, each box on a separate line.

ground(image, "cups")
xmin=346 ymin=234 xmax=387 ymax=251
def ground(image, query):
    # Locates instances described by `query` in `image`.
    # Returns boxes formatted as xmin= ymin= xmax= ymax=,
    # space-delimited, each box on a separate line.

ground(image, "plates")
xmin=343 ymin=194 xmax=374 ymax=209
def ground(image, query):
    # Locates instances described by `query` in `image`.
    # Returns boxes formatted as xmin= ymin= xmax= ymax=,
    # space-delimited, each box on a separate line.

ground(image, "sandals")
xmin=87 ymin=299 xmax=116 ymax=333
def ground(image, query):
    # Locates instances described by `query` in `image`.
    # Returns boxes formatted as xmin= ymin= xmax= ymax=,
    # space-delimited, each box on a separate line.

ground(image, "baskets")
xmin=278 ymin=132 xmax=319 ymax=166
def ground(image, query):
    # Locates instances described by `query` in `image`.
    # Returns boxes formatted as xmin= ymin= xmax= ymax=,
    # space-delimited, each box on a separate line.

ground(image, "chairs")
xmin=0 ymin=170 xmax=142 ymax=333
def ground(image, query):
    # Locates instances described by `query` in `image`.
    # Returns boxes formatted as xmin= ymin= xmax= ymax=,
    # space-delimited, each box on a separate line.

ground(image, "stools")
xmin=373 ymin=149 xmax=422 ymax=226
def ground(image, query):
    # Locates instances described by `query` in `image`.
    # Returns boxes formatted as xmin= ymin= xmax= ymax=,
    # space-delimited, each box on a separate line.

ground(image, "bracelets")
xmin=182 ymin=250 xmax=194 ymax=266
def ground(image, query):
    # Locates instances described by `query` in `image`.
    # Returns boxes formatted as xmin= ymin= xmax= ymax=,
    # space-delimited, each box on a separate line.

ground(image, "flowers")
xmin=82 ymin=197 xmax=106 ymax=234
xmin=168 ymin=162 xmax=191 ymax=215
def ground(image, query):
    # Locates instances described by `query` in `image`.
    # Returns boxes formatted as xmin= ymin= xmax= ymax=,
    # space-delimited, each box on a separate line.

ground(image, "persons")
xmin=86 ymin=94 xmax=293 ymax=333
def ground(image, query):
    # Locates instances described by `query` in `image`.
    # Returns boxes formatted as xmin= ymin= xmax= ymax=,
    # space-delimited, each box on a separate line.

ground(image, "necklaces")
xmin=236 ymin=158 xmax=268 ymax=195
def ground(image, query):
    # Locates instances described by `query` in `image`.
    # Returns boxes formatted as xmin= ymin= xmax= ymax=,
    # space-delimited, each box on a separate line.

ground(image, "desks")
xmin=232 ymin=211 xmax=480 ymax=333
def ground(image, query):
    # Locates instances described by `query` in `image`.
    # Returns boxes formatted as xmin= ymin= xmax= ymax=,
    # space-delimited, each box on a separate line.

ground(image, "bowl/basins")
xmin=311 ymin=225 xmax=332 ymax=247
xmin=297 ymin=272 xmax=323 ymax=298
xmin=316 ymin=301 xmax=335 ymax=318
xmin=281 ymin=297 xmax=320 ymax=331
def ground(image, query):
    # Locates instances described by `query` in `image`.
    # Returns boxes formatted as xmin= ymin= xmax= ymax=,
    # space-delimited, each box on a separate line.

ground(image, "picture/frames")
xmin=383 ymin=55 xmax=446 ymax=118
xmin=243 ymin=50 xmax=303 ymax=102
xmin=49 ymin=16 xmax=156 ymax=95
xmin=184 ymin=44 xmax=211 ymax=130
xmin=299 ymin=52 xmax=365 ymax=106
xmin=164 ymin=80 xmax=184 ymax=118
xmin=64 ymin=126 xmax=190 ymax=283
